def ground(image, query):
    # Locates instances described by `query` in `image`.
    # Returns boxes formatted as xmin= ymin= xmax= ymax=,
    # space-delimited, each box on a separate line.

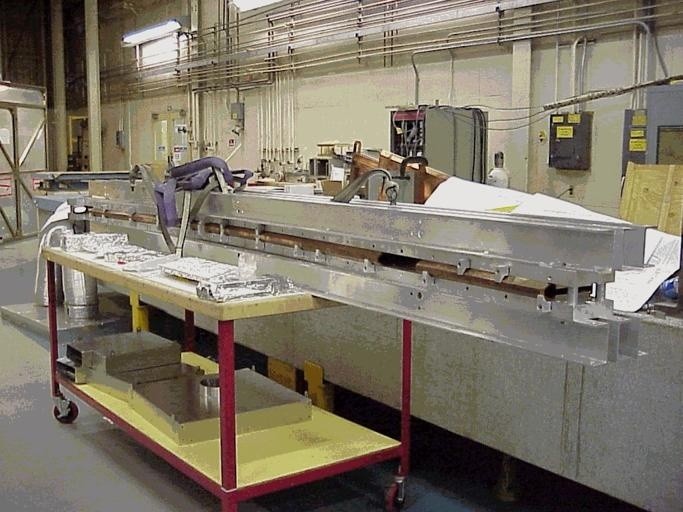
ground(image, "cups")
xmin=238 ymin=252 xmax=256 ymax=280
